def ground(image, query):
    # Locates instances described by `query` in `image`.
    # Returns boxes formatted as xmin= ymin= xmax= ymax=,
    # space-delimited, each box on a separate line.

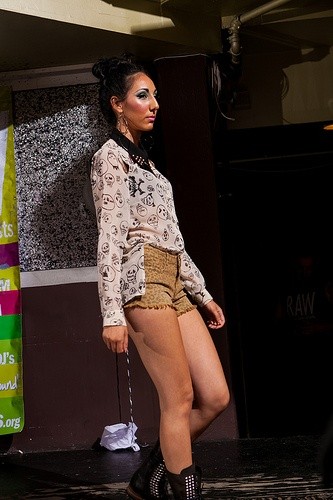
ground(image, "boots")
xmin=127 ymin=439 xmax=173 ymax=500
xmin=165 ymin=459 xmax=202 ymax=500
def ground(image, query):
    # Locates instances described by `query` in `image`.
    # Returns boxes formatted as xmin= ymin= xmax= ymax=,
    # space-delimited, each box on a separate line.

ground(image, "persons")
xmin=91 ymin=63 xmax=231 ymax=500
